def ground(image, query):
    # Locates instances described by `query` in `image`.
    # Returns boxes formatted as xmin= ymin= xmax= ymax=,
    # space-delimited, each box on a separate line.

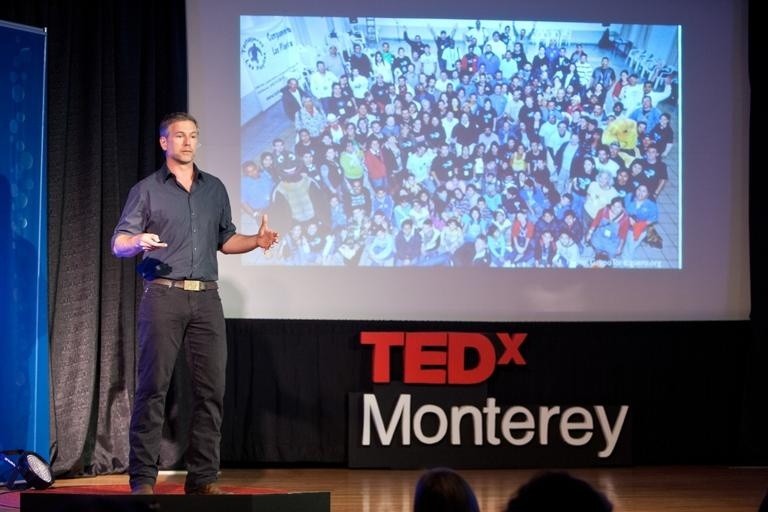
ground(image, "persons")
xmin=109 ymin=111 xmax=278 ymax=495
xmin=414 ymin=467 xmax=480 ymax=512
xmin=496 ymin=468 xmax=615 ymax=512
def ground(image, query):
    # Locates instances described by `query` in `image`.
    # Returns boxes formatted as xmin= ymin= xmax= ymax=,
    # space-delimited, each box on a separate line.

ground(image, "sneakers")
xmin=130 ymin=483 xmax=154 ymax=496
xmin=184 ymin=482 xmax=233 ymax=495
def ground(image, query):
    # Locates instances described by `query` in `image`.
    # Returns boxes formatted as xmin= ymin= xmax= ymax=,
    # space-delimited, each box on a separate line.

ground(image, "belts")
xmin=145 ymin=276 xmax=219 ymax=292
